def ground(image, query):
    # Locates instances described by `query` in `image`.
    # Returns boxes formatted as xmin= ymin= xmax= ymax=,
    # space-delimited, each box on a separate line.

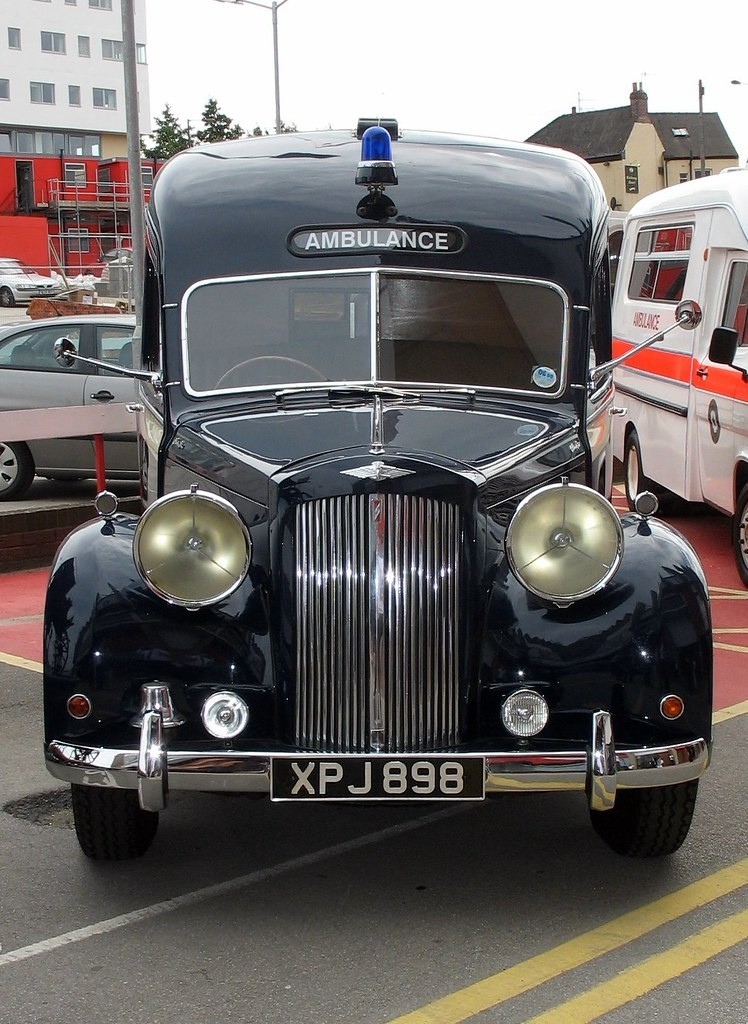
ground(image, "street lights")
xmin=218 ymin=0 xmax=290 ymax=134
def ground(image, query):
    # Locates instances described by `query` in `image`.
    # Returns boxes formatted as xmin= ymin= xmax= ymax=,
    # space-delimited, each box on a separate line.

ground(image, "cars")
xmin=0 ymin=257 xmax=63 ymax=307
xmin=40 ymin=116 xmax=715 ymax=859
xmin=83 ymin=246 xmax=135 ymax=277
xmin=0 ymin=315 xmax=158 ymax=502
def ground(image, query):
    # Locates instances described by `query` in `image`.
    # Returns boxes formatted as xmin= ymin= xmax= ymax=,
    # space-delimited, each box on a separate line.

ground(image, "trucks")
xmin=608 ymin=162 xmax=748 ymax=588
